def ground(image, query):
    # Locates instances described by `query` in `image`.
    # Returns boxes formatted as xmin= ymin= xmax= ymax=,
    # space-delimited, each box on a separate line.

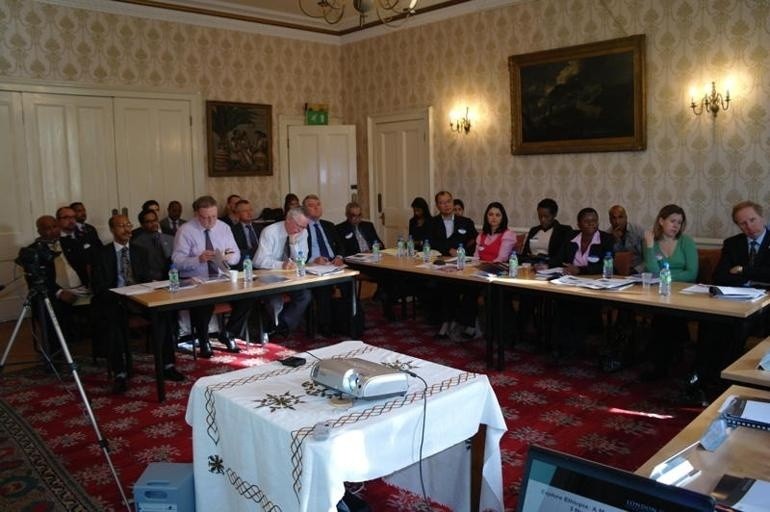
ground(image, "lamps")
xmin=687 ymin=77 xmax=735 ymax=118
xmin=449 ymin=103 xmax=472 ymax=136
xmin=294 ymin=0 xmax=424 ymax=35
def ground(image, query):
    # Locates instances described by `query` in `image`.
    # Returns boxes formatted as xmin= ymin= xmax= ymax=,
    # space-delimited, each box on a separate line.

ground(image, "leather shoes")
xmin=196 ymin=330 xmax=213 ymax=357
xmin=433 ymin=332 xmax=449 ymax=340
xmin=154 ymin=362 xmax=185 ymax=381
xmin=114 ymin=370 xmax=129 ymax=393
xmin=462 ymin=331 xmax=475 ymax=338
xmin=218 ymin=327 xmax=241 ymax=351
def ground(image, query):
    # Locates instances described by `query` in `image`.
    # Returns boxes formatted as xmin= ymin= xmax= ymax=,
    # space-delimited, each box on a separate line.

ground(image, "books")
xmin=711 ymin=348 xmax=769 ymax=511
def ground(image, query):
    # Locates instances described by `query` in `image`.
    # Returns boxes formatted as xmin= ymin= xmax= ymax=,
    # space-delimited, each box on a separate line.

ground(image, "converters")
xmin=282 ymin=356 xmax=306 ymax=367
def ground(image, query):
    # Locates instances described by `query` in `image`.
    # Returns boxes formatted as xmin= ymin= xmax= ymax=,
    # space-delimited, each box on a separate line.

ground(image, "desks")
xmin=182 ymin=340 xmax=496 ymax=512
xmin=720 ymin=338 xmax=769 ymax=389
xmin=490 ymin=262 xmax=767 ymax=399
xmin=637 ymin=384 xmax=768 ymax=511
xmin=111 ymin=259 xmax=359 ymax=404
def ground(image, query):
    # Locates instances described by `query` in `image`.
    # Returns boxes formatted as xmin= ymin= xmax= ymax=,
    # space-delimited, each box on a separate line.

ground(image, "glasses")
xmin=113 ymin=223 xmax=133 ymax=228
xmin=59 ymin=215 xmax=76 ymax=220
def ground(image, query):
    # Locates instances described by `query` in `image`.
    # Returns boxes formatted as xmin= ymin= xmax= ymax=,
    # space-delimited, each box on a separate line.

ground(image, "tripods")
xmin=0 ymin=270 xmax=132 ymax=512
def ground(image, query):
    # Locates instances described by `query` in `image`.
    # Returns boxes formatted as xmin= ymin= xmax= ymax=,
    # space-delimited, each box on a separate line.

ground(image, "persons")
xmin=18 ymin=193 xmax=385 ymax=382
xmin=230 ymin=130 xmax=242 ymax=169
xmin=397 ymin=191 xmax=698 ymax=383
xmin=251 ymin=130 xmax=268 ymax=171
xmin=693 ymin=201 xmax=770 ymax=376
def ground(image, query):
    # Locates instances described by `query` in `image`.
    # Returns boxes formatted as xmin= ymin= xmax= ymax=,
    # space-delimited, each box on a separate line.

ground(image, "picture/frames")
xmin=506 ymin=30 xmax=650 ymax=157
xmin=205 ymin=95 xmax=275 ymax=179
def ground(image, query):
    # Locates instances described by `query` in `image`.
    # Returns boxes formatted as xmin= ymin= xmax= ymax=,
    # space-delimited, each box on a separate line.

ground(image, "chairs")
xmin=350 ymin=245 xmax=529 ymax=381
xmin=359 ymin=258 xmax=768 ymax=368
xmin=20 ymin=249 xmax=349 ymax=375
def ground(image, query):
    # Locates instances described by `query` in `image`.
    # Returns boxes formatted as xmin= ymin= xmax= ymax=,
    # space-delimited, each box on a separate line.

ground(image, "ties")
xmin=51 ymin=244 xmax=70 ymax=289
xmin=747 ymin=241 xmax=757 ymax=273
xmin=282 ymin=236 xmax=290 ymax=261
xmin=204 ymin=228 xmax=219 ymax=279
xmin=313 ymin=224 xmax=330 ymax=261
xmin=245 ymin=225 xmax=258 ymax=250
xmin=172 ymin=220 xmax=177 ymax=233
xmin=120 ymin=247 xmax=134 ymax=286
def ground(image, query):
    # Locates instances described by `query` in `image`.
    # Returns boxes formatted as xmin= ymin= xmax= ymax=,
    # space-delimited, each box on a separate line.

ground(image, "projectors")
xmin=310 ymin=359 xmax=408 ymax=397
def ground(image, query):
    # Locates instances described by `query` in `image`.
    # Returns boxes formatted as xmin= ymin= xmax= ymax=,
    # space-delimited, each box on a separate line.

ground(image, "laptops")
xmin=512 ymin=442 xmax=716 ymax=512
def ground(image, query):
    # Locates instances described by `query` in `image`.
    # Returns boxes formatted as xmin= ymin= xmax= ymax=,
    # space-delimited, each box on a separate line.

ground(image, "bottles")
xmin=456 ymin=243 xmax=466 ymax=271
xmin=168 ymin=265 xmax=179 ymax=291
xmin=372 ymin=240 xmax=379 ymax=264
xmin=659 ymin=263 xmax=672 ymax=297
xmin=508 ymin=250 xmax=519 ymax=278
xmin=423 ymin=239 xmax=431 ymax=264
xmin=295 ymin=251 xmax=306 ymax=277
xmin=242 ymin=254 xmax=254 ymax=284
xmin=602 ymin=252 xmax=614 ymax=279
xmin=396 ymin=234 xmax=415 ymax=260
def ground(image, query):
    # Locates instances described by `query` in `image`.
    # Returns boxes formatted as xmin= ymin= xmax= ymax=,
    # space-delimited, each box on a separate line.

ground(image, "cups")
xmin=521 ymin=263 xmax=531 ymax=276
xmin=641 ymin=271 xmax=653 ymax=289
xmin=228 ymin=269 xmax=239 ymax=284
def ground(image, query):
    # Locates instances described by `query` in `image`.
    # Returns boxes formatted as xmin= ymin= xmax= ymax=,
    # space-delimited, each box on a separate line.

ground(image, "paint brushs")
xmin=225 ymin=250 xmax=235 ymax=254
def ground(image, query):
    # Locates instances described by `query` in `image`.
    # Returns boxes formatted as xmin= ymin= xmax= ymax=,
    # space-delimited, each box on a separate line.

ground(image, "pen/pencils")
xmin=288 ymin=258 xmax=292 ymax=261
